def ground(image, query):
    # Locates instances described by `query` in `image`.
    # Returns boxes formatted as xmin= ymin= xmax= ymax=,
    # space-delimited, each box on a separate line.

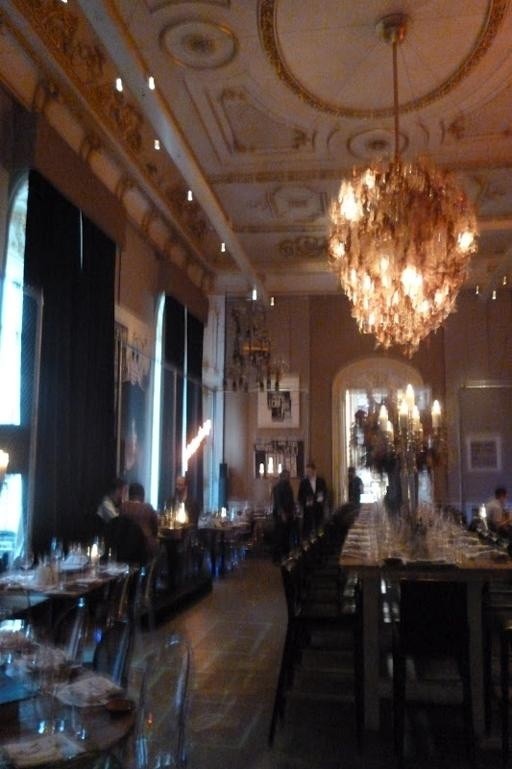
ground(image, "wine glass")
xmin=20 ymin=536 xmax=118 ymax=579
xmin=1 ymin=623 xmax=95 ymax=739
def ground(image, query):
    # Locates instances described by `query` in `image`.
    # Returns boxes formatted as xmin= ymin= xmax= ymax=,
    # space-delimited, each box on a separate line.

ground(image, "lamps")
xmin=329 ymin=14 xmax=478 ymax=360
xmin=379 ymin=382 xmax=443 ymax=533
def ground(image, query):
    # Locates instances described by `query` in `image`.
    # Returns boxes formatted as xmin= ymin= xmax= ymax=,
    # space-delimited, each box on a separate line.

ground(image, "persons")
xmin=347 ymin=466 xmax=365 ymax=505
xmin=116 ymin=483 xmax=158 ymax=560
xmin=486 ymin=487 xmax=512 ymax=529
xmin=269 ymin=468 xmax=298 ymax=567
xmin=298 ymin=464 xmax=328 ymax=544
xmin=94 ymin=478 xmax=128 ymax=534
xmin=160 ymin=474 xmax=197 ymax=528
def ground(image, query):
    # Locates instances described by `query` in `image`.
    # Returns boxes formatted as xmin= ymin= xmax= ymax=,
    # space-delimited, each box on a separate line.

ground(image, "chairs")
xmin=46 ymin=555 xmax=195 ymax=769
